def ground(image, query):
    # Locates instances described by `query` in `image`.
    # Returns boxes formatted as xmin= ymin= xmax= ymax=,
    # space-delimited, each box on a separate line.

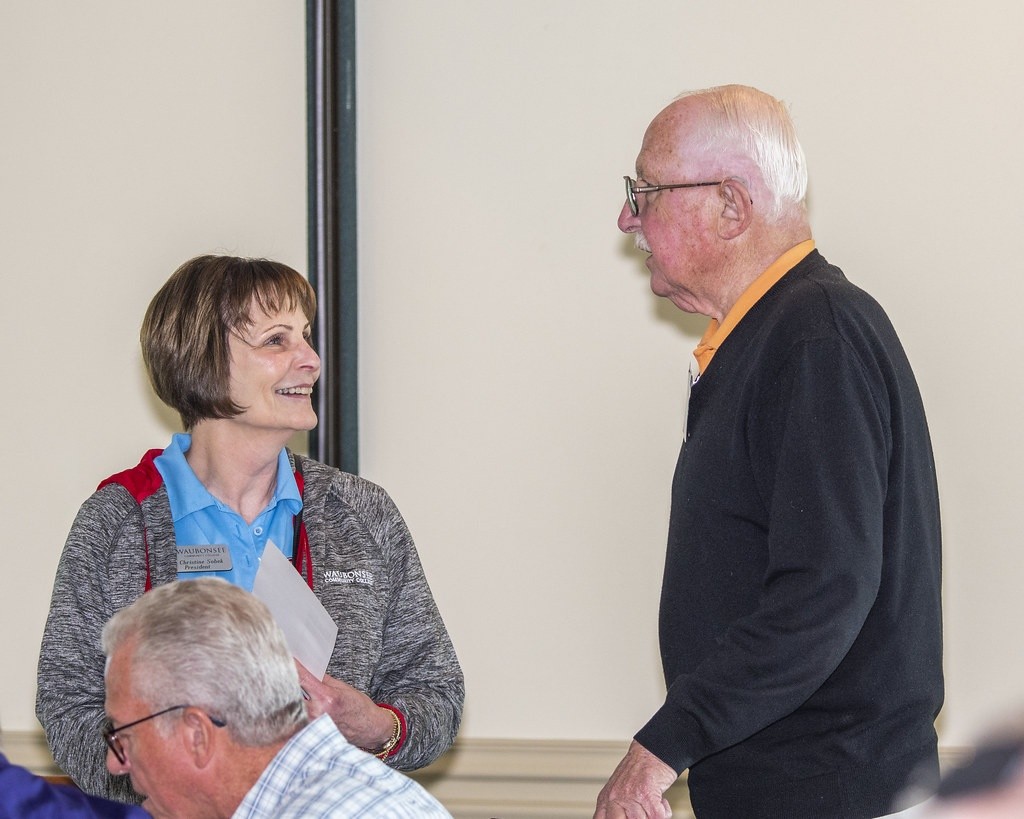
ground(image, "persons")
xmin=101 ymin=577 xmax=454 ymax=819
xmin=592 ymin=84 xmax=944 ymax=819
xmin=36 ymin=255 xmax=465 ymax=805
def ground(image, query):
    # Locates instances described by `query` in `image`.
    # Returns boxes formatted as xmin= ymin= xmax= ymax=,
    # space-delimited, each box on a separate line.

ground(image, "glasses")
xmin=624 ymin=175 xmax=752 ymax=219
xmin=102 ymin=703 xmax=228 ymax=765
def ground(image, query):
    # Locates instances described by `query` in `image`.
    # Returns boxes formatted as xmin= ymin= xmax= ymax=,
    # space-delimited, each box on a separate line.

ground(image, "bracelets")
xmin=367 ymin=708 xmax=401 ymax=762
